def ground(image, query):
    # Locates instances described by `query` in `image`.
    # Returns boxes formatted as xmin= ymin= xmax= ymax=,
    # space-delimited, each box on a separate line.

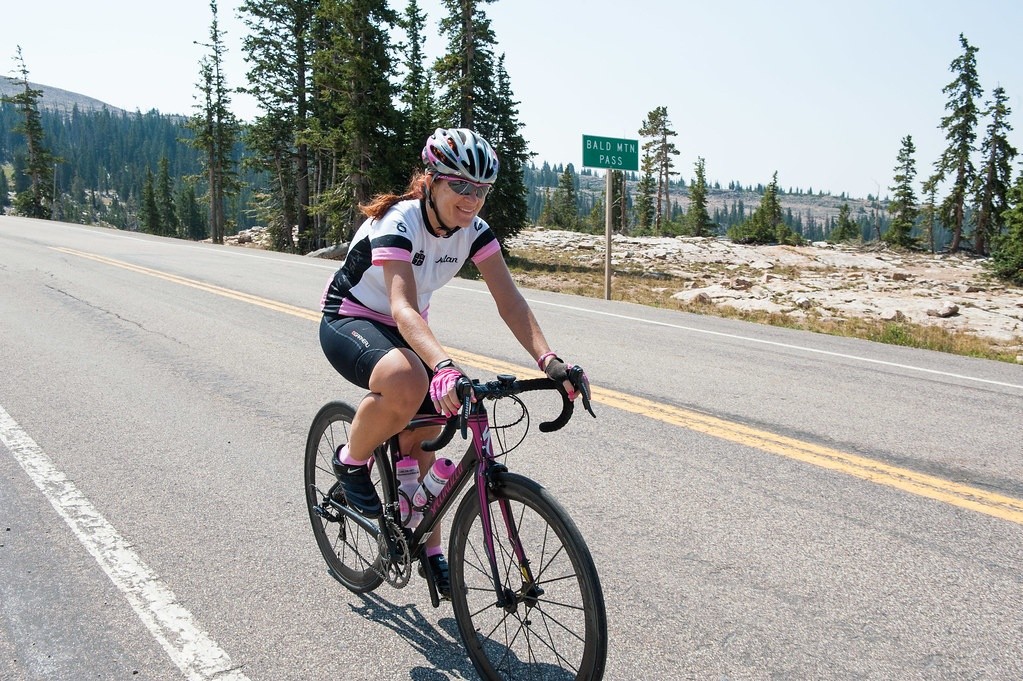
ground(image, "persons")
xmin=319 ymin=127 xmax=591 ymax=599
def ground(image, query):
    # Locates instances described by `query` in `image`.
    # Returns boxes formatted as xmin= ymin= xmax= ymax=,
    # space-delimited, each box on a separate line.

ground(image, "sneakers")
xmin=418 ymin=554 xmax=468 ymax=598
xmin=331 ymin=445 xmax=383 ymax=519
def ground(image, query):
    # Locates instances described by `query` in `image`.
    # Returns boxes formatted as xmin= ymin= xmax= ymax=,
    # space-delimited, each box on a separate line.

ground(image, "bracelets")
xmin=432 ymin=359 xmax=461 ymax=374
xmin=537 ymin=351 xmax=559 ymax=371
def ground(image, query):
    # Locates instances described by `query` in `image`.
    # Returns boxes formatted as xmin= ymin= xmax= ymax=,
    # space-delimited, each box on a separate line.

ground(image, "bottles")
xmin=413 ymin=458 xmax=455 ymax=510
xmin=396 ymin=456 xmax=424 ymax=528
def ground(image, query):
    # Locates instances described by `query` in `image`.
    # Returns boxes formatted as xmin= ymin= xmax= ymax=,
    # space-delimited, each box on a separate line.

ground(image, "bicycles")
xmin=303 ymin=365 xmax=609 ymax=681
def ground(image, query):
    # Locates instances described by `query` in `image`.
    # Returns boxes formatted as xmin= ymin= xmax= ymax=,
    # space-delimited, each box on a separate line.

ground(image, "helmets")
xmin=421 ymin=128 xmax=499 ymax=184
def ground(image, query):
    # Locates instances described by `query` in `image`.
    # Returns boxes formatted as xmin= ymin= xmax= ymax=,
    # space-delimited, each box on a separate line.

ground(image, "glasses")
xmin=436 ymin=175 xmax=492 ymax=199
